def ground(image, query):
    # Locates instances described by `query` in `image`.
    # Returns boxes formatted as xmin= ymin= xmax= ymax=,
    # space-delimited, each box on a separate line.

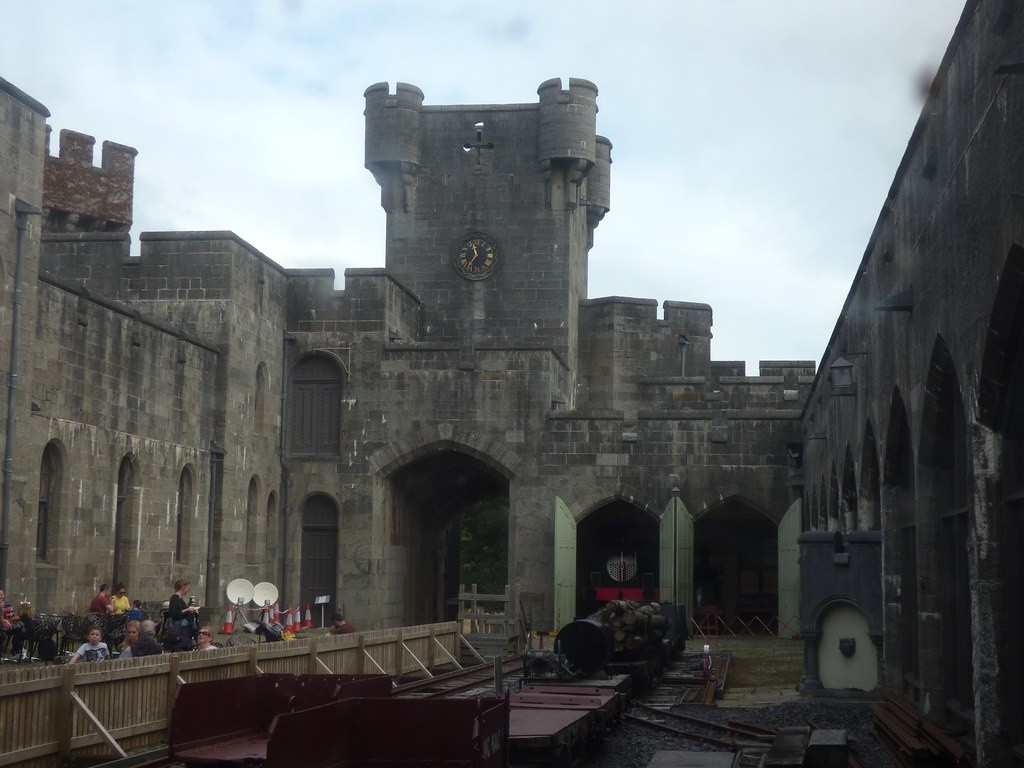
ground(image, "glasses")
xmin=119 ymin=591 xmax=126 ymax=595
xmin=200 ymin=632 xmax=211 ymax=637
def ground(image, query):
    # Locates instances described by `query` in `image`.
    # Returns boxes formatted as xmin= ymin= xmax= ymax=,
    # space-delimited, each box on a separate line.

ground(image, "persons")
xmin=123 ymin=600 xmax=142 ymax=621
xmin=91 ymin=584 xmax=115 ymax=615
xmin=168 ymin=579 xmax=198 ymax=641
xmin=330 ymin=615 xmax=354 ymax=634
xmin=0 ymin=589 xmax=37 ymax=660
xmin=69 ymin=627 xmax=110 ymax=663
xmin=198 ymin=627 xmax=217 ymax=650
xmin=117 ymin=620 xmax=164 ymax=659
xmin=110 ymin=583 xmax=130 ymax=616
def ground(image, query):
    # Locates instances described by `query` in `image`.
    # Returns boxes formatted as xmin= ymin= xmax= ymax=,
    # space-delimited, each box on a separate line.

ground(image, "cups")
xmin=190 ymin=596 xmax=195 ymax=604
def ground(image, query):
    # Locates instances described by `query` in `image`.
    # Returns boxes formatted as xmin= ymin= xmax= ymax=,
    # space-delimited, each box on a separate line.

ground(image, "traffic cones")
xmin=283 ymin=608 xmax=294 ymax=634
xmin=293 ymin=605 xmax=302 ymax=632
xmin=303 ymin=601 xmax=314 ymax=629
xmin=262 ymin=601 xmax=281 ymax=625
xmin=219 ymin=604 xmax=237 ymax=634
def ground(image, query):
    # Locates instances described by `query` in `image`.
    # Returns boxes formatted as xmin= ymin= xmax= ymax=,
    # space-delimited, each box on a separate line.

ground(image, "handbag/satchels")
xmin=166 ymin=627 xmax=182 ymax=642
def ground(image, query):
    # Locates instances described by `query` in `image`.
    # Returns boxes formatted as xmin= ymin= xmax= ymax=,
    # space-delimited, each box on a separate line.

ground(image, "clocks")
xmin=450 ymin=230 xmax=504 ymax=281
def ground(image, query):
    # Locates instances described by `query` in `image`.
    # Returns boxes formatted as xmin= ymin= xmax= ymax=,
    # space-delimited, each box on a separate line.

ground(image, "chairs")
xmin=0 ymin=609 xmax=258 ymax=666
